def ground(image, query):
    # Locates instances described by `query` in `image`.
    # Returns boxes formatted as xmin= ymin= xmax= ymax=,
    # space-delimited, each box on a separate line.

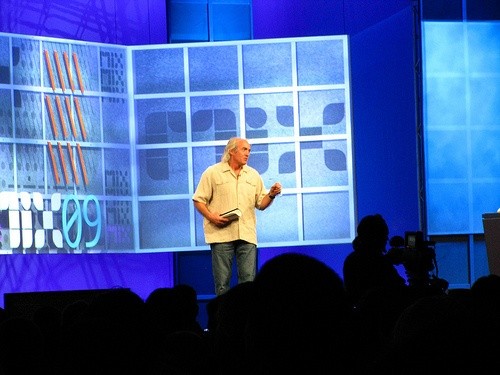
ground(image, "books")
xmin=220 ymin=208 xmax=242 ymax=221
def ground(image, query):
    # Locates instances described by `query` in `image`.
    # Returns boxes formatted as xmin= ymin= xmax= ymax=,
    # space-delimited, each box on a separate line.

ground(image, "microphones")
xmin=390 ymin=236 xmax=406 ymax=248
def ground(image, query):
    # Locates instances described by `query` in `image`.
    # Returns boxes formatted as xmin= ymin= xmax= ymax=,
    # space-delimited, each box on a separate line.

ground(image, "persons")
xmin=192 ymin=137 xmax=282 ymax=295
xmin=0 ymin=214 xmax=500 ymax=375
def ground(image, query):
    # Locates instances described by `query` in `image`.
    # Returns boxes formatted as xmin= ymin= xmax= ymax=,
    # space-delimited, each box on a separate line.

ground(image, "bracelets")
xmin=268 ymin=193 xmax=275 ymax=199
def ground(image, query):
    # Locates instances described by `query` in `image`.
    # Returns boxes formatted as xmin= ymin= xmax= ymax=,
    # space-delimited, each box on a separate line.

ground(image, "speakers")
xmin=3 ymin=288 xmax=143 ymax=313
